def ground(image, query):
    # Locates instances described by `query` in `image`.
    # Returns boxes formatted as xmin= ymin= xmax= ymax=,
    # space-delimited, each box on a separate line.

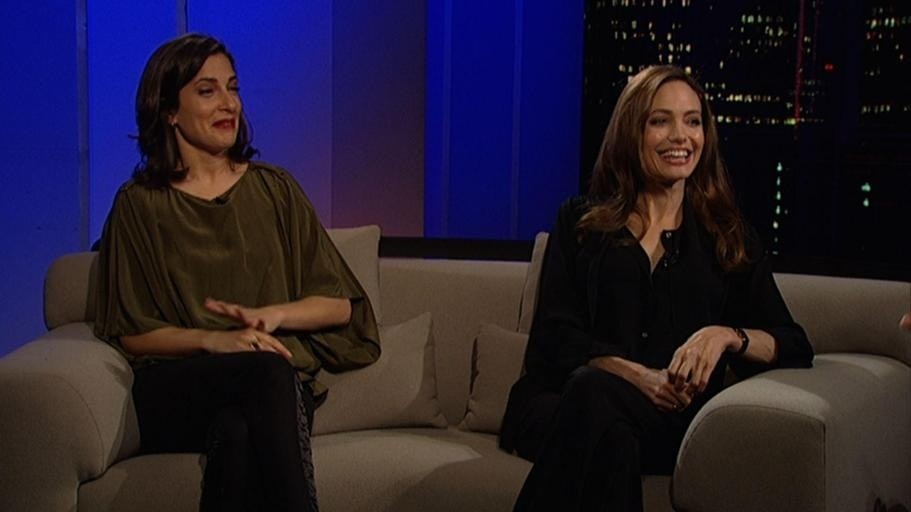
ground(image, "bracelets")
xmin=731 ymin=325 xmax=749 ymax=357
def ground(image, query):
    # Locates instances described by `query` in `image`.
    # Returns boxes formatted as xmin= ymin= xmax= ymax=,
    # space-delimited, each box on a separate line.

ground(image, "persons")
xmin=498 ymin=64 xmax=815 ymax=512
xmin=92 ymin=33 xmax=385 ymax=510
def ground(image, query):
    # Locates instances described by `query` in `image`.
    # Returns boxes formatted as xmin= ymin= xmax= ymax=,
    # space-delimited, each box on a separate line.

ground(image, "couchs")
xmin=0 ymin=251 xmax=909 ymax=512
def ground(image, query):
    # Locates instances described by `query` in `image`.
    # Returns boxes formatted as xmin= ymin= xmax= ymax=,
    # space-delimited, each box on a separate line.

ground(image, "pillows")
xmin=311 ymin=312 xmax=450 ymax=435
xmin=457 ymin=319 xmax=529 ymax=434
xmin=324 ymin=224 xmax=384 ymax=326
xmin=516 ymin=232 xmax=550 ymax=332
